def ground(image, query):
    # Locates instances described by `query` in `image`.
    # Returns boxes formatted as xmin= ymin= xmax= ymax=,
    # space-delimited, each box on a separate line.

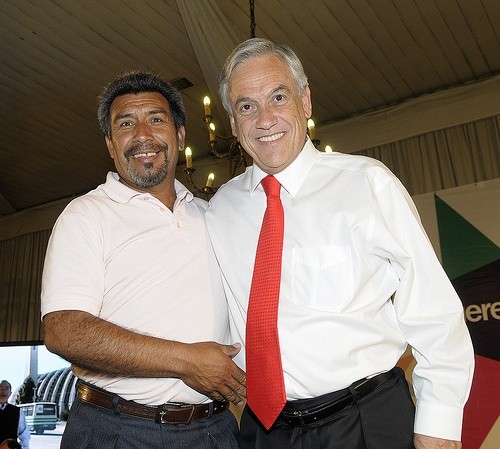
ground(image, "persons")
xmin=40 ymin=70 xmax=247 ymax=449
xmin=203 ymin=38 xmax=476 ymax=449
xmin=0 ymin=380 xmax=31 ymax=449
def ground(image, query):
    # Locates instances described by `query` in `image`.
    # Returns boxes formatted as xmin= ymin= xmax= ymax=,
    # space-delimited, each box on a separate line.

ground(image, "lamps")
xmin=183 ymin=0 xmax=333 ymax=194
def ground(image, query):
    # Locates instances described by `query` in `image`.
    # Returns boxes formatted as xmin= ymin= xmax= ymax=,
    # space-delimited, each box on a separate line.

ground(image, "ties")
xmin=246 ymin=175 xmax=289 ymax=430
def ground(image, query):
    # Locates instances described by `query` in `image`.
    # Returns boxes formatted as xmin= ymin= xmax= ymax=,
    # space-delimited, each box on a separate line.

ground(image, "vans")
xmin=15 ymin=402 xmax=57 ymax=434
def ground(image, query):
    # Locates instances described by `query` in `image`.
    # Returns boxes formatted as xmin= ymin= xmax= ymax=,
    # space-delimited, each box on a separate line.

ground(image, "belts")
xmin=272 ymin=365 xmax=401 ymax=428
xmin=74 ymin=382 xmax=231 ymax=425
